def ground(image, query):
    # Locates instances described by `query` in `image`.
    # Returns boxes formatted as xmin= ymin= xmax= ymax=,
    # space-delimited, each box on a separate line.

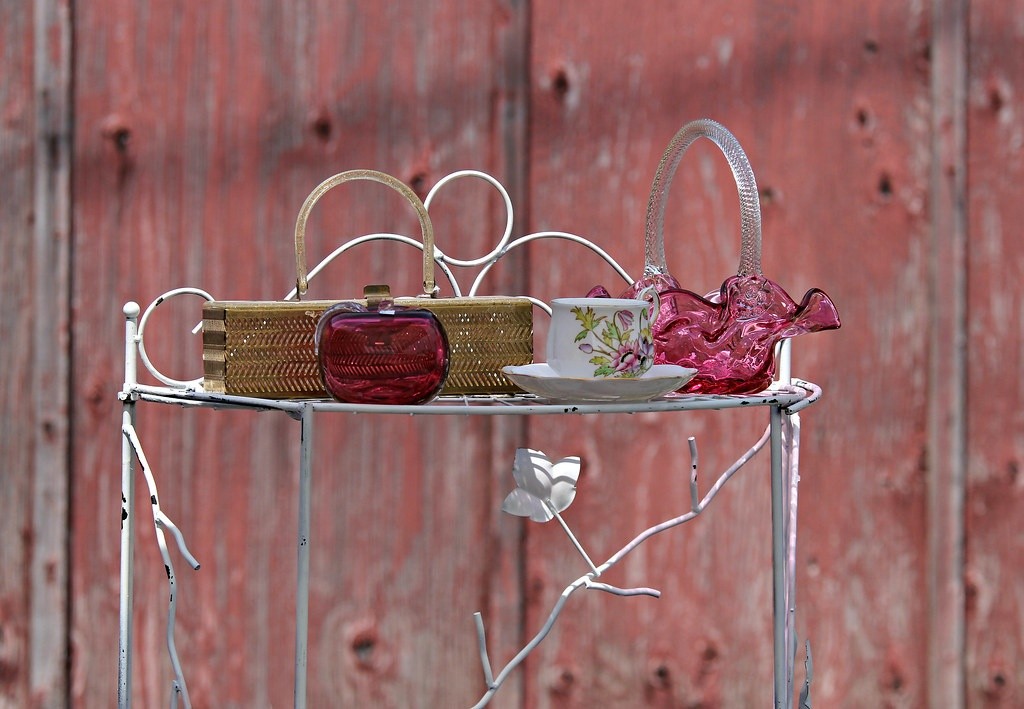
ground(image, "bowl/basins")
xmin=584 ymin=274 xmax=842 ymax=396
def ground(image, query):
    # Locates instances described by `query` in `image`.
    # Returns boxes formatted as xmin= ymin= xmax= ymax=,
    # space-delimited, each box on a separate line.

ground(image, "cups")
xmin=546 ymin=286 xmax=661 ymax=379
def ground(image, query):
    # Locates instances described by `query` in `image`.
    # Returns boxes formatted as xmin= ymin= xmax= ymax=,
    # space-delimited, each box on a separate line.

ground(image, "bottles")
xmin=315 ymin=299 xmax=451 ymax=408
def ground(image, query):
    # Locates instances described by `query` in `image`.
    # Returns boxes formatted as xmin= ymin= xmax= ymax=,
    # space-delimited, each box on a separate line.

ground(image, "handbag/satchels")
xmin=202 ymin=170 xmax=534 ymax=404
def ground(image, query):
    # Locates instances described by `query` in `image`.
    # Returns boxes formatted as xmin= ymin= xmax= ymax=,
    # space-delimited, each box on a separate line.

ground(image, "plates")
xmin=501 ymin=362 xmax=699 ymax=401
